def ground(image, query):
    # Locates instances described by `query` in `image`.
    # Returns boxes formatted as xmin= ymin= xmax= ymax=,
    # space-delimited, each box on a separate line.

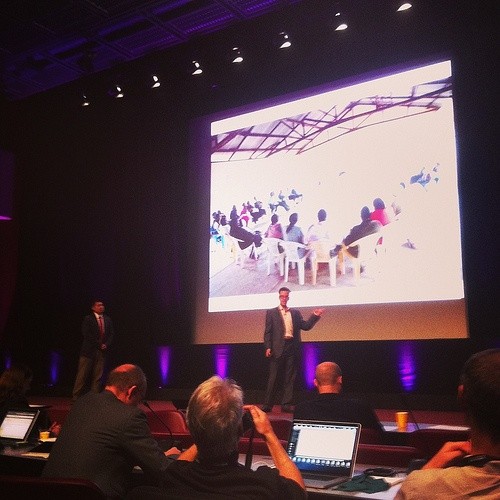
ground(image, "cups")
xmin=396 ymin=412 xmax=408 ymax=431
xmin=40 ymin=432 xmax=50 ymax=440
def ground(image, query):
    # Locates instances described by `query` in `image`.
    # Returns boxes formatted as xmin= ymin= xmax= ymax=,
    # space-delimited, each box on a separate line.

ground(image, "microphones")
xmin=142 ymin=400 xmax=173 ymax=442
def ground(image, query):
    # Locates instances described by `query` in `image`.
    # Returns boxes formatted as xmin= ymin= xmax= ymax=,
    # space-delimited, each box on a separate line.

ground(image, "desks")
xmin=238 ymin=453 xmax=409 ymax=500
xmin=378 ymin=421 xmax=471 ymax=446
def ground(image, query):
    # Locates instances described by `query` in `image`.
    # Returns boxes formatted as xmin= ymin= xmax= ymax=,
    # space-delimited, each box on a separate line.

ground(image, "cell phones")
xmin=363 ymin=468 xmax=393 ymax=477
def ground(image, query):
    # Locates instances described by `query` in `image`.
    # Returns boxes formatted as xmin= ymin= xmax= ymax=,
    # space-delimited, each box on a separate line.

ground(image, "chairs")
xmin=356 ymin=428 xmax=423 ymax=468
xmin=147 ymin=411 xmax=192 ymax=442
xmin=285 ymin=241 xmax=309 ymax=285
xmin=0 ymin=475 xmax=108 ymax=500
xmin=217 ymin=224 xmax=257 ymax=268
xmin=238 ymin=418 xmax=293 ymax=454
xmin=310 ymin=240 xmax=338 ymax=287
xmin=262 ymin=237 xmax=284 ymax=276
xmin=340 ymin=231 xmax=384 ymax=281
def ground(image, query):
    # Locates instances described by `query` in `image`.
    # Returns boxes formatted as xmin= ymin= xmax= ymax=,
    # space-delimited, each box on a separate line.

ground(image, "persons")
xmin=210 ymin=160 xmax=442 ymax=275
xmin=292 ymin=360 xmax=385 ymax=430
xmin=259 ymin=288 xmax=327 ymax=415
xmin=39 ymin=362 xmax=180 ymax=500
xmin=392 ymin=348 xmax=500 ymax=500
xmin=0 ymin=369 xmax=63 ymax=439
xmin=143 ymin=375 xmax=307 ymax=500
xmin=69 ymin=298 xmax=110 ymax=398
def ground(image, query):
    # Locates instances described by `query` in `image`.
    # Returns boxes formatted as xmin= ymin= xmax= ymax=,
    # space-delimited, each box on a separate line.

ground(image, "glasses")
xmin=279 ymin=295 xmax=290 ymax=297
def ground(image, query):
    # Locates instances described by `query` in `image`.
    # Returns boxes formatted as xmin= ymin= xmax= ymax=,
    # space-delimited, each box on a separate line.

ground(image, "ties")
xmin=99 ymin=316 xmax=103 ymax=338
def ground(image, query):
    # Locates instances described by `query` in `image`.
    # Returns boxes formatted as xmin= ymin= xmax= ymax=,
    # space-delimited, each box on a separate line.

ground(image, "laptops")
xmin=0 ymin=409 xmax=40 ymax=446
xmin=285 ymin=419 xmax=362 ymax=489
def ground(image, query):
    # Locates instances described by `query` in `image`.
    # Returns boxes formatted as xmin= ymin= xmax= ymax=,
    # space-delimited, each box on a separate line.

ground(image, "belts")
xmin=283 ymin=336 xmax=293 ymax=340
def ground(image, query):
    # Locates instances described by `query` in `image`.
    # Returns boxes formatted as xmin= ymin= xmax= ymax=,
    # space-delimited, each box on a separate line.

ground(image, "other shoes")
xmin=281 ymin=403 xmax=297 ymax=413
xmin=249 ymin=253 xmax=260 ymax=259
xmin=259 ymin=404 xmax=272 ymax=412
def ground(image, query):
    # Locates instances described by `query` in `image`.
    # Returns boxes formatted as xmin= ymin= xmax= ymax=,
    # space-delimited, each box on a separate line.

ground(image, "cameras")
xmin=241 ymin=409 xmax=254 ymax=433
